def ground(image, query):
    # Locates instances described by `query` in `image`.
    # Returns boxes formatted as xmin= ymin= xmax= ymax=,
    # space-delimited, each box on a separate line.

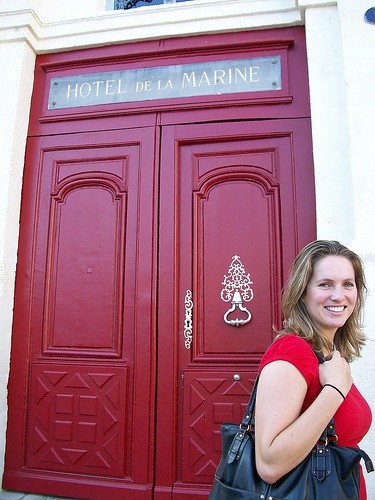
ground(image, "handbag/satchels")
xmin=203 ymin=335 xmax=374 ymax=499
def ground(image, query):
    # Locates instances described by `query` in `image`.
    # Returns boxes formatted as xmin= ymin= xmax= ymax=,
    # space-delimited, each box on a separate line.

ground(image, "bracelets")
xmin=322 ymin=384 xmax=347 ymax=401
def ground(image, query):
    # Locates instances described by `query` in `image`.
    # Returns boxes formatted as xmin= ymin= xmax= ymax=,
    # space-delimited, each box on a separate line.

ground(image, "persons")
xmin=253 ymin=238 xmax=374 ymax=500
